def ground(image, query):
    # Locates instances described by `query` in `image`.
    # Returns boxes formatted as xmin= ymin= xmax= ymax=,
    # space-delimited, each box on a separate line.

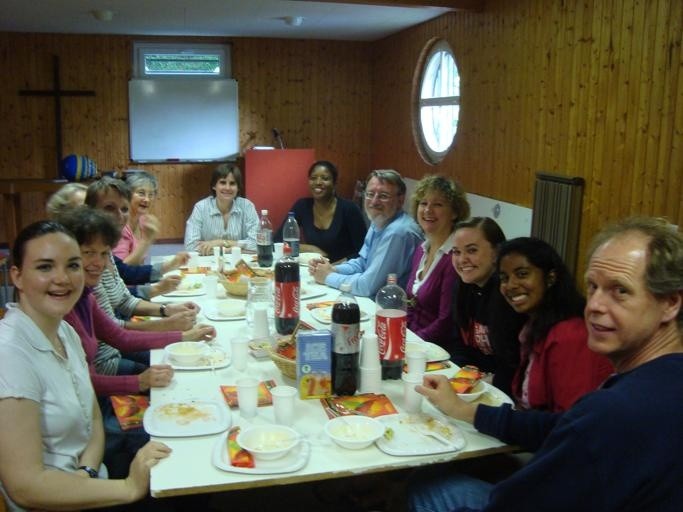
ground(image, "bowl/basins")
xmin=326 ymin=415 xmax=383 ymax=450
xmin=236 ymin=424 xmax=297 ymax=462
xmin=166 ymin=342 xmax=207 ymax=364
xmin=454 ymin=380 xmax=488 ymax=402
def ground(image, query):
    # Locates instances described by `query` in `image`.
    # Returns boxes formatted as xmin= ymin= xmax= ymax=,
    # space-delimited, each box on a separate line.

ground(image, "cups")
xmin=236 ymin=378 xmax=258 ymax=416
xmin=408 ymin=352 xmax=426 ymax=375
xmin=274 ymin=243 xmax=283 ymax=258
xmin=403 ymin=375 xmax=425 ymax=412
xmin=270 ymin=386 xmax=297 ymax=425
xmin=186 ymin=245 xmax=242 ymax=265
xmin=358 ymin=334 xmax=384 ymax=394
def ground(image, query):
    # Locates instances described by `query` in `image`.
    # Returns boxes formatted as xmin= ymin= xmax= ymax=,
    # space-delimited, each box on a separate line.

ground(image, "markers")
xmin=165 ymin=159 xmax=179 ymax=162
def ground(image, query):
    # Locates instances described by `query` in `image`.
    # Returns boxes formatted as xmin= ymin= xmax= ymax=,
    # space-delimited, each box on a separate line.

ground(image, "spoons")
xmin=416 ymin=424 xmax=459 ymax=451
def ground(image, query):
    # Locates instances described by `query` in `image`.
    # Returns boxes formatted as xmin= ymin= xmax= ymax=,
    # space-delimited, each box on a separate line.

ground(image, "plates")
xmin=373 ymin=414 xmax=466 ymax=457
xmin=168 ymin=346 xmax=232 ymax=370
xmin=407 ymin=341 xmax=450 ymax=364
xmin=311 ymin=305 xmax=370 ymax=325
xmin=211 ymin=429 xmax=310 ymax=475
xmin=302 ymin=282 xmax=328 ymax=300
xmin=163 ymin=288 xmax=205 ymax=296
xmin=205 ymin=300 xmax=244 ymax=321
xmin=143 ymin=400 xmax=232 ymax=437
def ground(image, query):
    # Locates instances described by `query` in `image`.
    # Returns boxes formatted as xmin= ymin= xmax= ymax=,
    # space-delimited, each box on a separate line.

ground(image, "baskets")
xmin=268 ymin=347 xmax=296 ymax=379
xmin=220 ymin=268 xmax=273 ymax=296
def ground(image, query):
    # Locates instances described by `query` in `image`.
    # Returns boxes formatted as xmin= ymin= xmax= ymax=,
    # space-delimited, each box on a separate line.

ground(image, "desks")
xmin=1 ymin=177 xmax=98 ymax=252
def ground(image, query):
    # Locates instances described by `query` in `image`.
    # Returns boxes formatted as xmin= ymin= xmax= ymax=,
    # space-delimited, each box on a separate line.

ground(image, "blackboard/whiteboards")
xmin=128 ymin=78 xmax=238 ymax=163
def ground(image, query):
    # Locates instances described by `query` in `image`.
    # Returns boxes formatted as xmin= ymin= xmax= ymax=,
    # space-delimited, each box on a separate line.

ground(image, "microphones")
xmin=272 ymin=128 xmax=280 ymax=138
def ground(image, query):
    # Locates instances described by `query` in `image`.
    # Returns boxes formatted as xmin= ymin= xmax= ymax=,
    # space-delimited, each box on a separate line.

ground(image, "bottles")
xmin=282 ymin=211 xmax=300 ymax=260
xmin=246 ymin=277 xmax=273 ymax=346
xmin=331 ymin=282 xmax=360 ymax=396
xmin=374 ymin=274 xmax=407 ymax=381
xmin=256 ymin=210 xmax=273 ymax=268
xmin=275 ymin=246 xmax=301 ymax=336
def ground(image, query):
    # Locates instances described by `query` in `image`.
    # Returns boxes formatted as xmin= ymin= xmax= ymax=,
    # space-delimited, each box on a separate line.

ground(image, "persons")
xmin=398 ymin=172 xmax=474 ymax=351
xmin=183 ymin=161 xmax=266 ymax=257
xmin=392 ymin=213 xmax=683 ymax=512
xmin=446 ymin=214 xmax=525 ymax=400
xmin=492 ymin=232 xmax=617 ymax=417
xmin=272 ymin=158 xmax=367 ymax=263
xmin=77 ymin=466 xmax=97 ymax=477
xmin=46 ymin=170 xmax=219 ymax=468
xmin=0 ymin=220 xmax=178 ymax=512
xmin=300 ymin=167 xmax=426 ymax=307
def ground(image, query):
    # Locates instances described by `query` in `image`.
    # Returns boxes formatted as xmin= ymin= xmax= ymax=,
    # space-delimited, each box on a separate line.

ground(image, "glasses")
xmin=365 ymin=191 xmax=392 ymax=202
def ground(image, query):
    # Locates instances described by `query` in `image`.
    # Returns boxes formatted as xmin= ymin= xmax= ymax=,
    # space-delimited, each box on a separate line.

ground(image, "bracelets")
xmin=224 ymin=240 xmax=230 ymax=248
xmin=301 ymin=243 xmax=305 ymax=253
